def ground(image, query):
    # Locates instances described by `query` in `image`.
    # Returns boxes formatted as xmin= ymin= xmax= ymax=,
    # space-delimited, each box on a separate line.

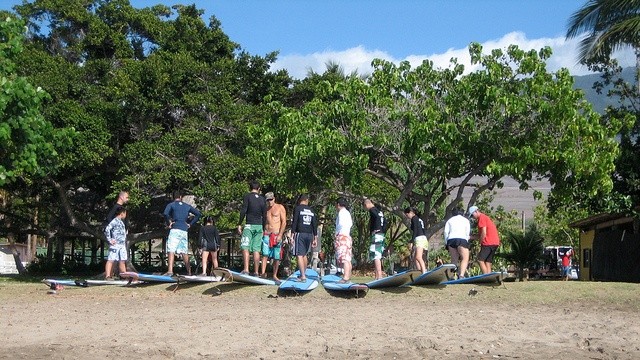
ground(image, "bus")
xmin=507 ymin=246 xmax=580 ymax=280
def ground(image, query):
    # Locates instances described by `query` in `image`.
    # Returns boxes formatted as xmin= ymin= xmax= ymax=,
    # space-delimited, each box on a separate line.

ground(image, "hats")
xmin=265 ymin=191 xmax=274 ymax=201
xmin=467 ymin=205 xmax=478 ymax=220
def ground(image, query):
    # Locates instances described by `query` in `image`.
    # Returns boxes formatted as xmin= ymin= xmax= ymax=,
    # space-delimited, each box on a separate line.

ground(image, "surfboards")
xmin=214 ymin=267 xmax=285 ymax=285
xmin=119 ymin=272 xmax=184 ymax=281
xmin=279 ymin=268 xmax=320 ymax=291
xmin=441 ymin=272 xmax=509 ymax=284
xmin=40 ymin=278 xmax=84 ymax=286
xmin=84 ymin=279 xmax=143 ymax=285
xmin=410 ymin=264 xmax=458 ymax=285
xmin=365 ymin=269 xmax=422 ymax=288
xmin=177 ymin=274 xmax=228 ymax=281
xmin=321 ymin=274 xmax=368 ymax=291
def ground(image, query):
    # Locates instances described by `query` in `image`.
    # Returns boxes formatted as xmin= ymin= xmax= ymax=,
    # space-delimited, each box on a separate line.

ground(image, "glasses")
xmin=266 ymin=199 xmax=273 ymax=201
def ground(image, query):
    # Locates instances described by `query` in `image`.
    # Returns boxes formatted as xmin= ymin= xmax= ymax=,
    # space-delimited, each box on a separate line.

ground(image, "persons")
xmin=362 ymin=198 xmax=387 ymax=281
xmin=162 ymin=191 xmax=201 ymax=276
xmin=443 ymin=207 xmax=471 ymax=279
xmin=467 ymin=205 xmax=500 ymax=274
xmin=435 ymin=255 xmax=443 ymax=268
xmin=291 ymin=194 xmax=317 ymax=282
xmin=558 ymin=249 xmax=574 ymax=281
xmin=237 ymin=180 xmax=266 ymax=276
xmin=197 ymin=215 xmax=221 ymax=276
xmin=333 ymin=197 xmax=353 ymax=284
xmin=106 ymin=191 xmax=129 ymax=235
xmin=103 ymin=207 xmax=128 ymax=281
xmin=260 ymin=191 xmax=287 ymax=282
xmin=403 ymin=207 xmax=429 ymax=274
